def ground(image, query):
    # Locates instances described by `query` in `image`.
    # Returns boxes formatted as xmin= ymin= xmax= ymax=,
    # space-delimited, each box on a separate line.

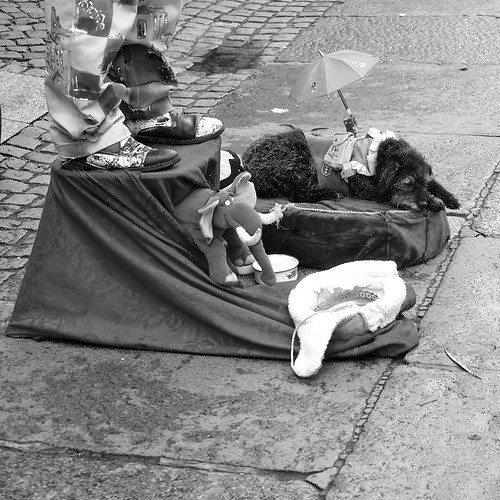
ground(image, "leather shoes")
xmin=126 ymin=111 xmax=225 ymax=144
xmin=61 ymin=136 xmax=181 ymax=173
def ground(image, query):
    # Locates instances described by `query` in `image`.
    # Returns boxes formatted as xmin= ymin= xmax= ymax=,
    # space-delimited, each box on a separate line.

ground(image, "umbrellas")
xmin=286 ymin=50 xmax=380 ymax=110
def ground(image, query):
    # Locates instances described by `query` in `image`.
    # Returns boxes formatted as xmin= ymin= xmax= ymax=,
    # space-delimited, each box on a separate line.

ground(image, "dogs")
xmin=239 ymin=127 xmax=463 ymax=212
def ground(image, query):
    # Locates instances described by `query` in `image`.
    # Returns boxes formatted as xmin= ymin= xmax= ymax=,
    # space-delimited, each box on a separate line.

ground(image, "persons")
xmin=45 ymin=0 xmax=225 ymax=172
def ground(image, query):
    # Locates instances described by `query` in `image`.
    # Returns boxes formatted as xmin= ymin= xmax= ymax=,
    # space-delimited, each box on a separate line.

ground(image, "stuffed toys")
xmin=174 ymin=171 xmax=281 ymax=286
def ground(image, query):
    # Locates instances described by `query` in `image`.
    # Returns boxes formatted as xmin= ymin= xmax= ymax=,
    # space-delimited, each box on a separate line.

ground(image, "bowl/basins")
xmin=252 ymin=254 xmax=299 ymax=284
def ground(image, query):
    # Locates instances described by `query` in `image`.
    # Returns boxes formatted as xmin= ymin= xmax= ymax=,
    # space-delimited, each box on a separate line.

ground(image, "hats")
xmin=287 ymin=260 xmax=415 ymax=379
